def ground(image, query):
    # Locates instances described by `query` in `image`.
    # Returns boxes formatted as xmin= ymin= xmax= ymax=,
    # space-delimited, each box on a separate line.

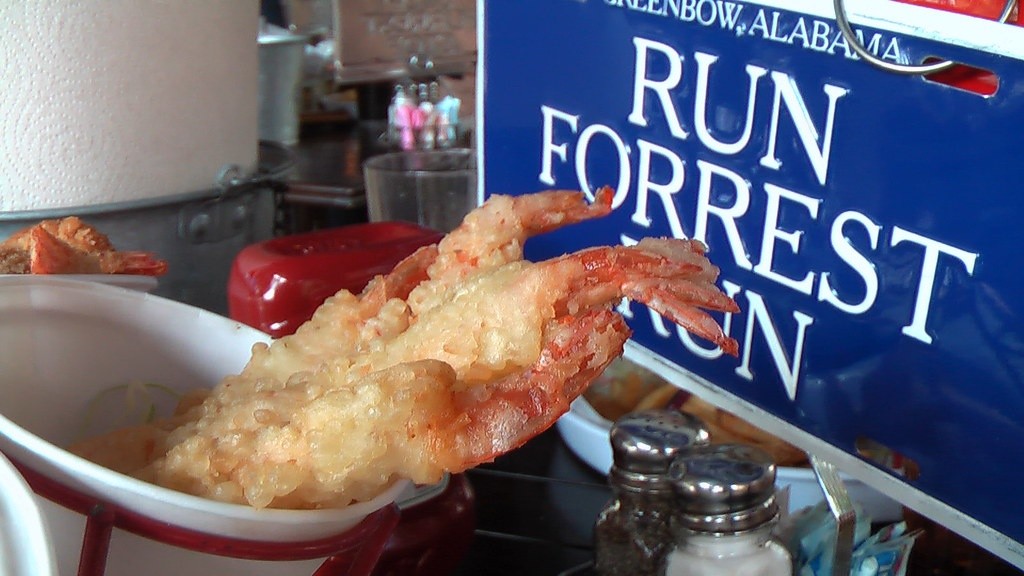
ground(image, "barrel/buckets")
xmin=258 ymin=35 xmax=311 ymax=144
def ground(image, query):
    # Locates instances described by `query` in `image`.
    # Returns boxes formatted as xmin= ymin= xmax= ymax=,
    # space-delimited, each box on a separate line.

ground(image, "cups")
xmin=0 ymin=274 xmax=407 ymax=576
xmin=362 ymin=148 xmax=479 ymax=233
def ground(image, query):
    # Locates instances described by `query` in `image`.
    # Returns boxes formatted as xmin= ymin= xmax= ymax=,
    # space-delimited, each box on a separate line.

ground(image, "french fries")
xmin=600 ymin=371 xmax=809 ymax=463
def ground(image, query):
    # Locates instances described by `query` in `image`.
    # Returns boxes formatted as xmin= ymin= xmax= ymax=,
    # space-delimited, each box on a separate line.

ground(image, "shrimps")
xmin=0 ymin=214 xmax=169 ymax=277
xmin=59 ymin=177 xmax=744 ymax=512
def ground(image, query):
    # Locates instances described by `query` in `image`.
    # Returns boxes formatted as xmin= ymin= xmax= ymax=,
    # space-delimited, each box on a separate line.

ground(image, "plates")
xmin=555 ymin=393 xmax=903 ymax=524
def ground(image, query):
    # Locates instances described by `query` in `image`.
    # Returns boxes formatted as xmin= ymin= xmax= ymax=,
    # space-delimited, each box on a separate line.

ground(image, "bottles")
xmin=664 ymin=442 xmax=794 ymax=576
xmin=593 ymin=404 xmax=711 ymax=575
xmin=229 ymin=222 xmax=476 ymax=576
